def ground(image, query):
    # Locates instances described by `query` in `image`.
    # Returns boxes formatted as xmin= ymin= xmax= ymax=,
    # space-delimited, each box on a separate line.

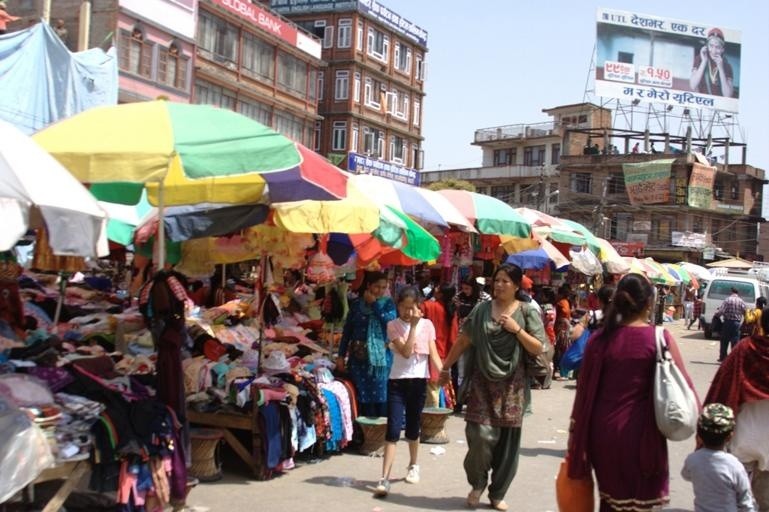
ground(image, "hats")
xmin=697 ymin=403 xmax=737 ymax=436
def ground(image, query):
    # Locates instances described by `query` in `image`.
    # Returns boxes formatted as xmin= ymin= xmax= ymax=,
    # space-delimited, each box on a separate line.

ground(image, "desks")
xmin=25 ymin=458 xmax=93 ymax=512
xmin=185 ymin=408 xmax=323 ymax=479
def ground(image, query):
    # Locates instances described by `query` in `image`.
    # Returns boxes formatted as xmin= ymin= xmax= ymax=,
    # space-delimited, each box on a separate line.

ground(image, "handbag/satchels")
xmin=559 ymin=327 xmax=590 ymax=370
xmin=653 ymin=326 xmax=699 ymax=442
xmin=520 ymin=304 xmax=554 ymax=391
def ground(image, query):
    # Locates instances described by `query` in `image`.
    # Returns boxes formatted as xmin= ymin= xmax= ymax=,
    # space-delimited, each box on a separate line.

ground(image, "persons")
xmin=337 ymin=264 xmax=769 ymax=511
xmin=690 ymin=28 xmax=734 ymax=99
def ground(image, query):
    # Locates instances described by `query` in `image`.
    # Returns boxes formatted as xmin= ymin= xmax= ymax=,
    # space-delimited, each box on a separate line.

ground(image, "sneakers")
xmin=403 ymin=463 xmax=420 ymax=484
xmin=467 ymin=481 xmax=490 ymax=507
xmin=490 ymin=494 xmax=510 ymax=510
xmin=373 ymin=473 xmax=391 ymax=495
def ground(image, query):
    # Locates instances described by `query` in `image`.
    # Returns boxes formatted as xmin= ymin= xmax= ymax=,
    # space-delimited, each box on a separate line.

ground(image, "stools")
xmin=355 ymin=415 xmax=388 ymax=458
xmin=189 ymin=428 xmax=224 ymax=482
xmin=419 ymin=407 xmax=455 ymax=445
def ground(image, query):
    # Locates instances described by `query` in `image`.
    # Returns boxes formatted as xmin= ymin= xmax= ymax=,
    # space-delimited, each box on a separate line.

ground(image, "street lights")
xmin=536 ymin=187 xmax=560 ymax=213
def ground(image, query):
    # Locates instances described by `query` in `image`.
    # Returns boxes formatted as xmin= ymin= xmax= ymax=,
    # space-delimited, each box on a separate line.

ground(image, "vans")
xmin=698 ymin=276 xmax=769 ymax=338
